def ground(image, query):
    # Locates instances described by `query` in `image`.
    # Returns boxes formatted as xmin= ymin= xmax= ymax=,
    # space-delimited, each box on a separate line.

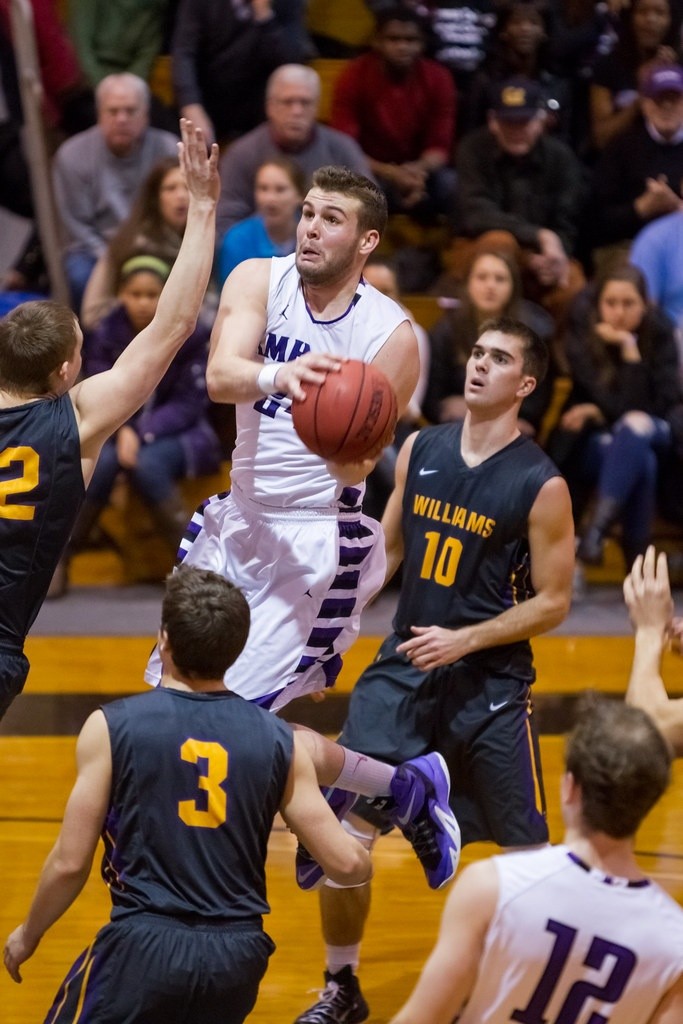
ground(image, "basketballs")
xmin=291 ymin=360 xmax=399 ymax=461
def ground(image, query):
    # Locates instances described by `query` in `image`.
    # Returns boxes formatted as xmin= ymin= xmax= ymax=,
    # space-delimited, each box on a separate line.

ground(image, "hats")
xmin=648 ymin=66 xmax=683 ymax=98
xmin=493 ymin=77 xmax=543 ymax=118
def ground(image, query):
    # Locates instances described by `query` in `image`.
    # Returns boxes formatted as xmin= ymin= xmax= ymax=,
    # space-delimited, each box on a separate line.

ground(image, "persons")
xmin=619 ymin=541 xmax=681 ymax=766
xmin=150 ymin=162 xmax=467 ymax=892
xmin=387 ymin=699 xmax=682 ymax=1024
xmin=0 ymin=1 xmax=683 ymax=608
xmin=3 ymin=567 xmax=373 ymax=1024
xmin=293 ymin=320 xmax=577 ymax=1024
xmin=0 ymin=120 xmax=220 ymax=718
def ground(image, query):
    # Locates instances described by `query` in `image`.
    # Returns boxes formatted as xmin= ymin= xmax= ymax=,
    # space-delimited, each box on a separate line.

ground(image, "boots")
xmin=621 ymin=540 xmax=650 ymax=574
xmin=144 ymin=489 xmax=190 ymax=562
xmin=576 ymin=496 xmax=620 ymax=566
xmin=43 ymin=499 xmax=109 ymax=599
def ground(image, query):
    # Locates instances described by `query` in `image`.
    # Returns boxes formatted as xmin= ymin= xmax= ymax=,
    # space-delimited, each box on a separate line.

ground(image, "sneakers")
xmin=294 ymin=965 xmax=369 ymax=1024
xmin=296 ymin=786 xmax=360 ymax=891
xmin=367 ymin=752 xmax=461 ymax=889
